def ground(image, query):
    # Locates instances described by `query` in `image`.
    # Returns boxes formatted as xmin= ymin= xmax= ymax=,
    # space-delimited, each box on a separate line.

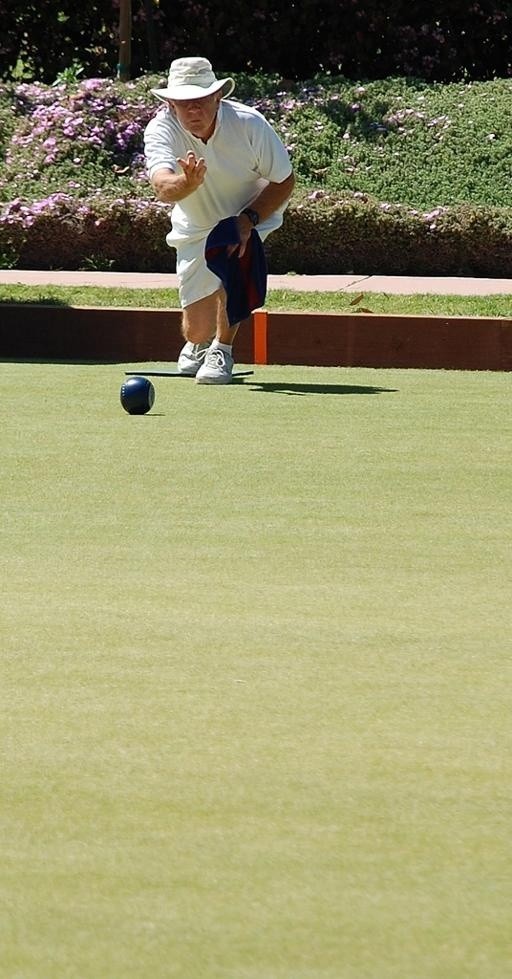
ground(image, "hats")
xmin=150 ymin=56 xmax=235 ymax=105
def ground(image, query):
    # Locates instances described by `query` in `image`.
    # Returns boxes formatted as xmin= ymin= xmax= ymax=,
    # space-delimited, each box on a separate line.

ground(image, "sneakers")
xmin=194 ymin=347 xmax=235 ymax=384
xmin=177 ymin=335 xmax=216 ymax=373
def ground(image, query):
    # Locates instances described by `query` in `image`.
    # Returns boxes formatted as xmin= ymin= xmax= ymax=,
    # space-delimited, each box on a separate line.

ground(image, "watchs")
xmin=239 ymin=208 xmax=261 ymax=227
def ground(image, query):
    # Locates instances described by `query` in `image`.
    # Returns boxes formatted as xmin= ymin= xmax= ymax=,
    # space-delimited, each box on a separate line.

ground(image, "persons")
xmin=141 ymin=56 xmax=298 ymax=387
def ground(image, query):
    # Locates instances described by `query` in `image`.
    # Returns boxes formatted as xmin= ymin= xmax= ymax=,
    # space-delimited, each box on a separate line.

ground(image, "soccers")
xmin=119 ymin=377 xmax=156 ymax=415
